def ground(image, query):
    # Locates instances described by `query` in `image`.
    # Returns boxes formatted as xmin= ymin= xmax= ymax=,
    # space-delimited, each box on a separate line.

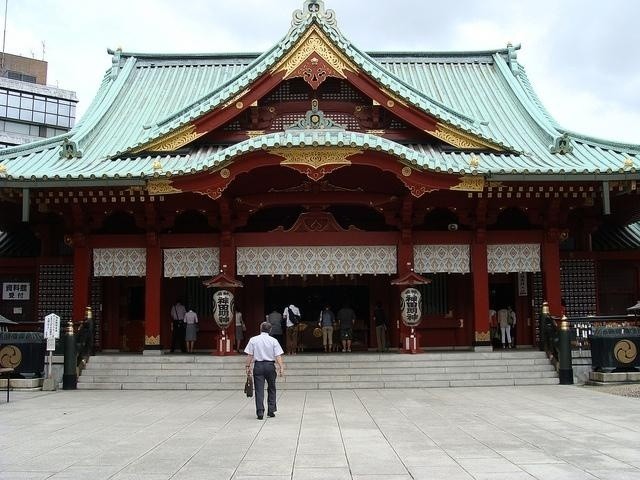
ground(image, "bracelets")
xmin=245 ymin=365 xmax=250 ymax=367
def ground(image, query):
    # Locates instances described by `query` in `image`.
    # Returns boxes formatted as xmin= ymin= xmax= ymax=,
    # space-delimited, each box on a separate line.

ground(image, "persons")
xmin=318 ymin=304 xmax=335 ymax=352
xmin=282 ymin=298 xmax=302 ymax=355
xmin=267 ymin=304 xmax=283 ymax=349
xmin=336 ymin=300 xmax=357 ymax=353
xmin=373 ymin=302 xmax=389 ymax=353
xmin=183 ymin=304 xmax=199 ymax=354
xmin=170 ymin=297 xmax=187 ymax=353
xmin=489 ymin=303 xmax=516 ymax=349
xmin=243 ymin=321 xmax=284 ymax=419
xmin=235 ymin=305 xmax=245 ymax=353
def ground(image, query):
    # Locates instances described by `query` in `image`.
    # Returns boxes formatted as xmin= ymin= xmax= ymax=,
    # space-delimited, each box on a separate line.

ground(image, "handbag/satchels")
xmin=244 ymin=375 xmax=253 ymax=397
xmin=491 ymin=315 xmax=497 ymax=327
xmin=507 ymin=316 xmax=512 ymax=325
xmin=288 ymin=309 xmax=300 ymax=325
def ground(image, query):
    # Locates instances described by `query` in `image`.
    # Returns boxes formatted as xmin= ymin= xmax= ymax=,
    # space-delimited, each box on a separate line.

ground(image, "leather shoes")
xmin=267 ymin=412 xmax=275 ymax=417
xmin=257 ymin=416 xmax=263 ymax=420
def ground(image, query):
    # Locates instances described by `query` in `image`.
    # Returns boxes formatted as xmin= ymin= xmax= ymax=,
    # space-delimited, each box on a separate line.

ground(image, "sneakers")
xmin=502 ymin=344 xmax=512 ymax=349
xmin=342 ymin=349 xmax=351 ymax=353
